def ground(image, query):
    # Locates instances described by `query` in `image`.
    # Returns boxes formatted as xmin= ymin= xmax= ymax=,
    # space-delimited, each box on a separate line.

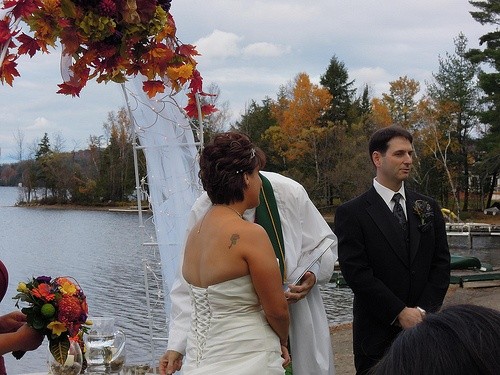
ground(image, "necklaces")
xmin=197 ymin=204 xmax=242 ymax=233
xmin=261 ymin=186 xmax=287 ymax=289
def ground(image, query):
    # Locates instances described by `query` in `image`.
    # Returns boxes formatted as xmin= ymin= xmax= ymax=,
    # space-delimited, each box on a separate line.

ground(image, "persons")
xmin=100 ymin=196 xmax=111 ymax=208
xmin=369 ymin=304 xmax=500 ymax=375
xmin=334 ymin=126 xmax=452 ymax=375
xmin=158 ymin=132 xmax=337 ymax=375
xmin=0 ymin=261 xmax=45 ymax=375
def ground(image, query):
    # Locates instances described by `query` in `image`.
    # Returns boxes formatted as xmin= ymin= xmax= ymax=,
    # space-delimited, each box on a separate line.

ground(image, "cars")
xmin=483 ymin=202 xmax=500 ymax=216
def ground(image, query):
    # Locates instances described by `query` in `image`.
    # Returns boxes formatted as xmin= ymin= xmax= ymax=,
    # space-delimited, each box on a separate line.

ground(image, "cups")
xmin=84 ymin=317 xmax=127 ymax=365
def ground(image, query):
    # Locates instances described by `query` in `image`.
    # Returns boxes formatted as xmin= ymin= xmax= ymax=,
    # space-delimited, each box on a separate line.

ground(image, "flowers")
xmin=10 ymin=274 xmax=89 ymax=364
xmin=0 ymin=0 xmax=219 ymax=119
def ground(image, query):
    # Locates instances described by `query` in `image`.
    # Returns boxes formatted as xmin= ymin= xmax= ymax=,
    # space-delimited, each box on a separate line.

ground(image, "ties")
xmin=391 ymin=193 xmax=410 ymax=255
